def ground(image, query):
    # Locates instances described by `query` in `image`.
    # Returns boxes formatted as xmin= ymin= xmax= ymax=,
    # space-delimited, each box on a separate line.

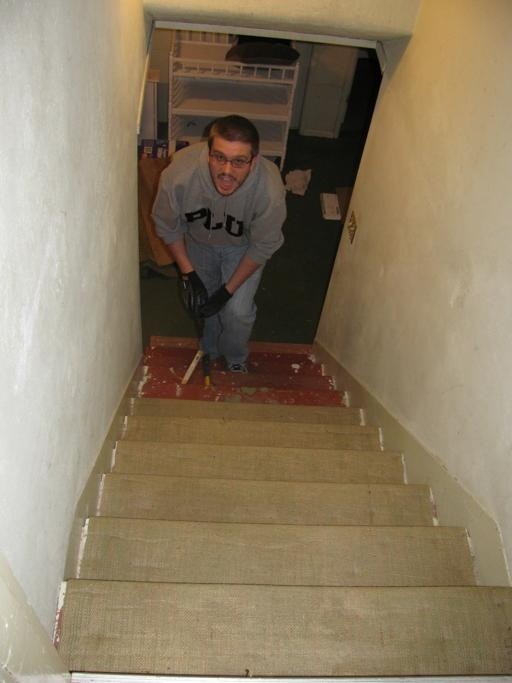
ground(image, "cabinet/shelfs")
xmin=166 ymin=32 xmax=300 ymax=174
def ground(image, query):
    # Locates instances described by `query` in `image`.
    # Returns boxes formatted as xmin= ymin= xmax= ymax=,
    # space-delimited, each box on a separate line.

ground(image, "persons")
xmin=151 ymin=112 xmax=288 ymax=373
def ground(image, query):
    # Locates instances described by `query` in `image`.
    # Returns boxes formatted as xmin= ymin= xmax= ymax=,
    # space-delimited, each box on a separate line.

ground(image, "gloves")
xmin=199 ymin=284 xmax=233 ymax=317
xmin=178 ymin=270 xmax=208 ymax=313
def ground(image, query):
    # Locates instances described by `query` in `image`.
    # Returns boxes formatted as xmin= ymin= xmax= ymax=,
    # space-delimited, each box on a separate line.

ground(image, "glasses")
xmin=209 ymin=148 xmax=252 ymax=168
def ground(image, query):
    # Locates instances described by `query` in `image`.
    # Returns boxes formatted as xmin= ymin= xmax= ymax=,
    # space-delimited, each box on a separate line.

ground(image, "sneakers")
xmin=230 ymin=364 xmax=243 ymax=373
xmin=208 ymin=356 xmax=226 ymax=375
